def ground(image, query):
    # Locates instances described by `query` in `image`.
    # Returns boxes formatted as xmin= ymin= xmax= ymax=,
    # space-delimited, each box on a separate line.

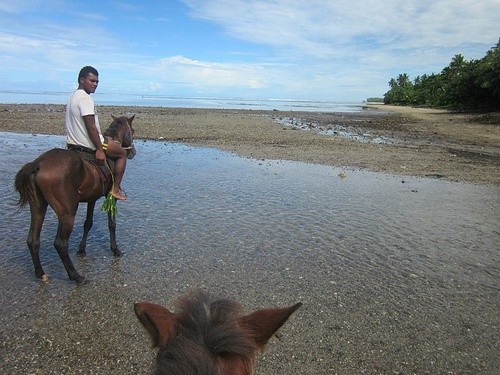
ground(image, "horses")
xmin=134 ymin=292 xmax=302 ymax=375
xmin=13 ymin=113 xmax=137 ymax=284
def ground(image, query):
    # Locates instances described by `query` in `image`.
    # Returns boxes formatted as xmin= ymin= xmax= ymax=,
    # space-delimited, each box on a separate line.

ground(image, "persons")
xmin=65 ymin=66 xmax=127 ymax=200
